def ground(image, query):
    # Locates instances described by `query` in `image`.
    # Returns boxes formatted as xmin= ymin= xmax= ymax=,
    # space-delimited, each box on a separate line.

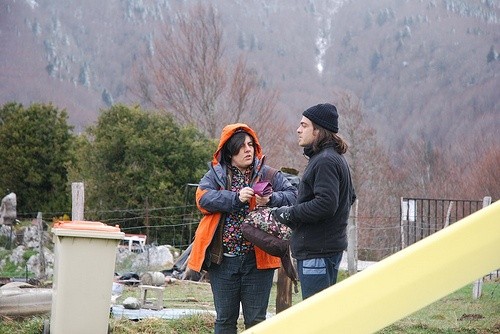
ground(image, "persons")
xmin=271 ymin=103 xmax=357 ymax=302
xmin=187 ymin=123 xmax=298 ymax=334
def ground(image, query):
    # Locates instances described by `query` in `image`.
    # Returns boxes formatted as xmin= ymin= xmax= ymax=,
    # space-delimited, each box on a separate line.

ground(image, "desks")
xmin=118 ymin=234 xmax=147 ymax=253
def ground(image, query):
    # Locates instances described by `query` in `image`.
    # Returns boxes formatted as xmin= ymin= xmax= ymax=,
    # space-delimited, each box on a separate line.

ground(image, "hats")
xmin=302 ymin=103 xmax=338 ymax=133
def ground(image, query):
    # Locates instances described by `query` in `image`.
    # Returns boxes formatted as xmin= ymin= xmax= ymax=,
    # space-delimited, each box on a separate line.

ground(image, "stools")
xmin=139 ymin=285 xmax=164 ymax=310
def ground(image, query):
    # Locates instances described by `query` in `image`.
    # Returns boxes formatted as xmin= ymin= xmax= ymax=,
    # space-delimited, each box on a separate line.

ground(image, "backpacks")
xmin=265 ymin=168 xmax=301 ymax=190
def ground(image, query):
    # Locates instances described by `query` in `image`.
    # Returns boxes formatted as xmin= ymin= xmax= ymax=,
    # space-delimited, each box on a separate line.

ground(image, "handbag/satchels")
xmin=242 ymin=208 xmax=299 ymax=292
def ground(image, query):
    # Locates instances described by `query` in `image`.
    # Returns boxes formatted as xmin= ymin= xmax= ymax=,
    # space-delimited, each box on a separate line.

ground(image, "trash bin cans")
xmin=50 ymin=221 xmax=125 ymax=334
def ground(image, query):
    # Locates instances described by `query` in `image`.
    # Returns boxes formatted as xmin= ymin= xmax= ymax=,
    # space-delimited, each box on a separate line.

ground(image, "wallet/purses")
xmin=253 ymin=181 xmax=273 ymax=196
